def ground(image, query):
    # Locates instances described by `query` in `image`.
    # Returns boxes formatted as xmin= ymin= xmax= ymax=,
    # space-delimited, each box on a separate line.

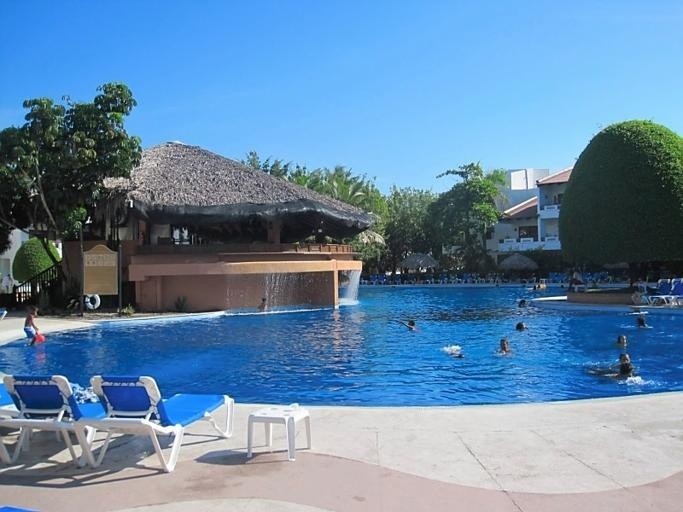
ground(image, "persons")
xmin=257 ymin=298 xmax=267 ymax=312
xmin=519 ymin=300 xmax=525 ymax=308
xmin=616 ymin=335 xmax=628 ymax=346
xmin=516 ymin=321 xmax=528 ymax=331
xmin=638 ymin=317 xmax=648 ymax=328
xmin=360 ymin=272 xmax=507 ymax=285
xmin=632 ymin=277 xmax=647 ymax=294
xmin=496 ymin=339 xmax=511 ymax=355
xmin=24 ymin=307 xmax=39 ymax=346
xmin=399 ymin=319 xmax=416 ymax=330
xmin=587 ymin=353 xmax=635 ymax=381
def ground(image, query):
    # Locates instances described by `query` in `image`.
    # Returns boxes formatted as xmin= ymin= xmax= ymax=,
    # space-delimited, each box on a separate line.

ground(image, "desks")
xmin=246 ymin=407 xmax=311 ymax=462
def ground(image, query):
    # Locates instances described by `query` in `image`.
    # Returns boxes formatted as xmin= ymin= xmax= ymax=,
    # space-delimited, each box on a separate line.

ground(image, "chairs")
xmin=0 ymin=372 xmax=235 ymax=473
xmin=638 ymin=278 xmax=683 ymax=307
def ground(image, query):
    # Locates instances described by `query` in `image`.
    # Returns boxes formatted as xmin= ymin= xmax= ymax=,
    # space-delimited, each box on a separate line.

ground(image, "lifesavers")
xmin=85 ymin=294 xmax=101 ymax=310
